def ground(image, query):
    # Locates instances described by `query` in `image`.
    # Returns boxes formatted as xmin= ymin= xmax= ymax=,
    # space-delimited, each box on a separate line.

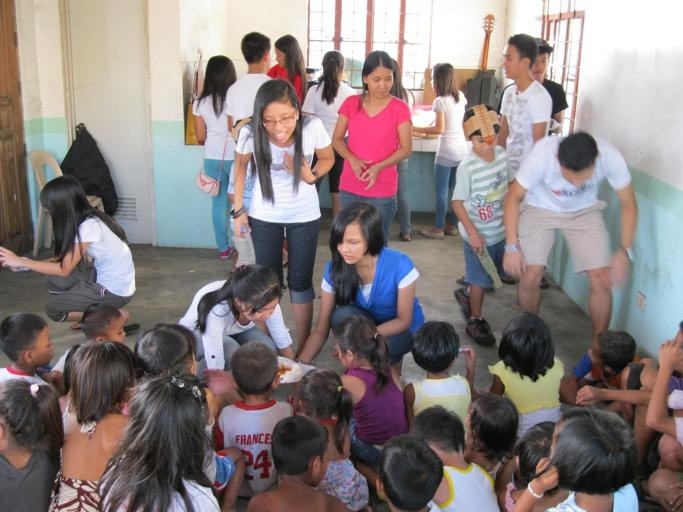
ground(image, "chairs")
xmin=28 ymin=149 xmax=105 ymax=258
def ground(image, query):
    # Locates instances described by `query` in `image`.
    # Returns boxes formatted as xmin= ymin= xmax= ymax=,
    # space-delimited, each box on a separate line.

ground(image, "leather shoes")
xmin=444 ymin=229 xmax=457 ymax=235
xmin=420 ymin=230 xmax=444 ymax=239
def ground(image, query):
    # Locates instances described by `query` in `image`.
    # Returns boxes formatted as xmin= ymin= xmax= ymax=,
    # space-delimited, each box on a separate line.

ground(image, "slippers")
xmin=71 ymin=321 xmax=81 ymax=329
xmin=122 ymin=324 xmax=138 ymax=332
xmin=399 ymin=232 xmax=410 ymax=241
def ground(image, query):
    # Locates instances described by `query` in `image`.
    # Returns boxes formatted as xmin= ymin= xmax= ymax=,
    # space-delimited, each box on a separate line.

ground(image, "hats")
xmin=534 ymin=37 xmax=553 ymax=56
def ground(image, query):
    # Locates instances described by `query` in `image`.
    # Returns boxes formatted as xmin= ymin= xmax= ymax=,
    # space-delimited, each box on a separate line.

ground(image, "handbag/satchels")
xmin=195 ymin=172 xmax=219 ymax=197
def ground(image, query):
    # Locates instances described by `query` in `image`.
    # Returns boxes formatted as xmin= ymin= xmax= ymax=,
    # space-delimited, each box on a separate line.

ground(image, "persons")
xmin=297 ymin=204 xmax=427 ymax=364
xmin=414 ymin=63 xmax=467 ymax=240
xmin=178 ymin=264 xmax=294 ymax=370
xmin=453 ymin=105 xmax=509 ymax=345
xmin=192 ymin=31 xmax=357 ymax=265
xmin=456 ymin=34 xmax=568 ymax=288
xmin=0 ymin=175 xmax=136 ymax=328
xmin=230 ymin=80 xmax=335 ymax=358
xmin=502 ymin=132 xmax=637 ymax=349
xmin=0 ymin=303 xmax=683 ymax=510
xmin=331 ymin=51 xmax=413 ymax=247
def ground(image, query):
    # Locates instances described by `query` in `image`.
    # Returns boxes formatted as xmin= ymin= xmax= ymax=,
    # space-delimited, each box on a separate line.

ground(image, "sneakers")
xmin=539 ymin=276 xmax=548 ymax=288
xmin=500 ymin=275 xmax=515 ymax=284
xmin=454 ymin=289 xmax=471 ymax=319
xmin=465 ymin=318 xmax=496 ymax=345
xmin=219 ymin=247 xmax=233 ymax=259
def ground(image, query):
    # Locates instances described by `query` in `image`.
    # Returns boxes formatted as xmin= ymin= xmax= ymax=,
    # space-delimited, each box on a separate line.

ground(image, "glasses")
xmin=261 ymin=109 xmax=295 ymax=127
